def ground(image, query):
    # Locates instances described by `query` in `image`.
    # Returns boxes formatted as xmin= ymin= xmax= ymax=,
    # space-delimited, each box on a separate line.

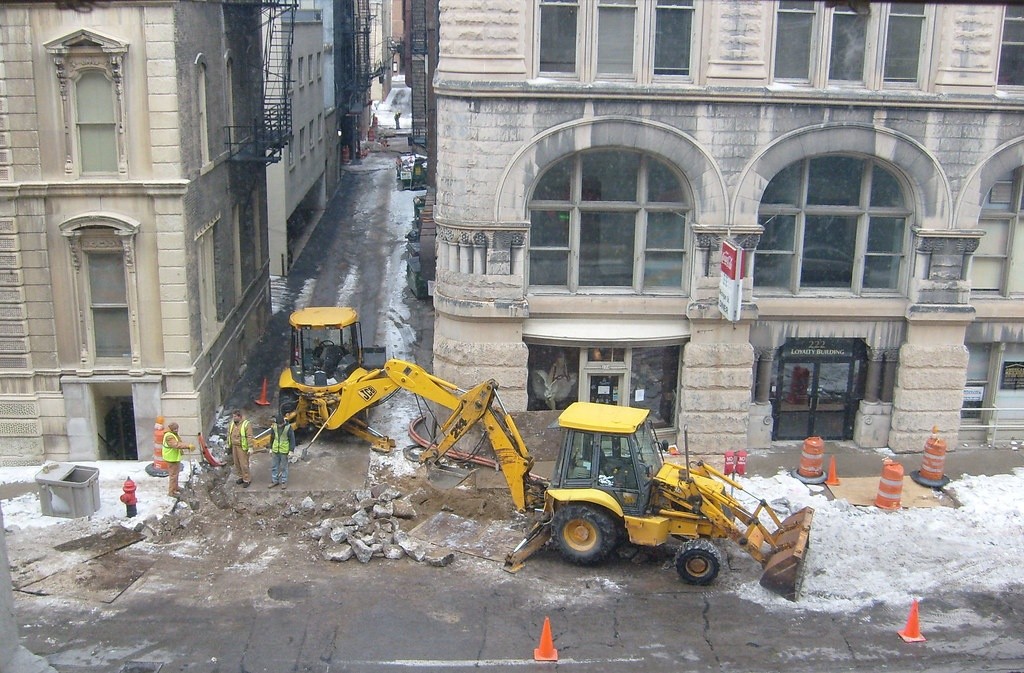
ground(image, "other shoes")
xmin=268 ymin=482 xmax=279 ymax=487
xmin=243 ymin=482 xmax=250 ymax=487
xmin=236 ymin=479 xmax=243 ymax=483
xmin=282 ymin=483 xmax=287 ymax=488
xmin=177 ymin=487 xmax=183 ymax=491
xmin=170 ymin=492 xmax=181 ymax=496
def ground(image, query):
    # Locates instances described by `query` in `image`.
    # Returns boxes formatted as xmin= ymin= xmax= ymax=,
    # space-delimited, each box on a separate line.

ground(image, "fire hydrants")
xmin=120 ymin=476 xmax=138 ymax=517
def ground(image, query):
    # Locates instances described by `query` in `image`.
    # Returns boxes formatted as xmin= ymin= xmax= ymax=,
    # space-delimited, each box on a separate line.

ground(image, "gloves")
xmin=288 ymin=451 xmax=294 ymax=458
xmin=189 ymin=444 xmax=195 ymax=451
xmin=247 ymin=447 xmax=254 ymax=455
xmin=270 ymin=449 xmax=272 ymax=454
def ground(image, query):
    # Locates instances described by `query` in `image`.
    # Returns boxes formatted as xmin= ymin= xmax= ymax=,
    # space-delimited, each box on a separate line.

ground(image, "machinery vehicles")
xmin=246 ymin=307 xmax=397 ymax=454
xmin=328 ymin=357 xmax=813 ymax=602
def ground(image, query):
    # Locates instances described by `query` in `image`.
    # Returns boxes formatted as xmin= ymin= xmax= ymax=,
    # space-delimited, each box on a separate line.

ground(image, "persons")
xmin=162 ymin=422 xmax=196 ymax=499
xmin=269 ymin=416 xmax=295 ymax=489
xmin=394 ymin=112 xmax=402 ymax=129
xmin=226 ymin=409 xmax=255 ymax=488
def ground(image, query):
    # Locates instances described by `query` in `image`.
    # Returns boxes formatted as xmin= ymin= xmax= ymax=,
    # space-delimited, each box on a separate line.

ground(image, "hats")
xmin=276 ymin=416 xmax=284 ymax=424
xmin=169 ymin=423 xmax=179 ymax=431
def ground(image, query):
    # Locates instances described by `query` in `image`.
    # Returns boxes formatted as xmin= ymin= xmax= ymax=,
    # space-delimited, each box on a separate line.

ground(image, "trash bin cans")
xmin=406 ymin=243 xmax=420 ymax=260
xmin=413 ymin=195 xmax=426 ymax=219
xmin=34 ymin=461 xmax=100 ymax=518
xmin=415 ymin=214 xmax=421 ymax=224
xmin=405 ymin=256 xmax=434 ymax=298
xmin=416 ymin=206 xmax=424 ymax=212
xmin=405 ymin=228 xmax=420 ymax=243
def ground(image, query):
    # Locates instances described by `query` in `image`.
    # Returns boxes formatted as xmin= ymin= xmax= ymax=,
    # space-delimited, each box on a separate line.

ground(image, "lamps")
xmin=825 ymin=0 xmax=871 ymax=15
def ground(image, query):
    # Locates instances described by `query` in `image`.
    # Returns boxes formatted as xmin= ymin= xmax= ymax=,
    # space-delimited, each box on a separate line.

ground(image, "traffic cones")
xmin=898 ymin=598 xmax=925 ymax=642
xmin=533 ymin=617 xmax=559 ymax=662
xmin=254 ymin=378 xmax=270 ymax=405
xmin=825 ymin=455 xmax=839 ymax=486
